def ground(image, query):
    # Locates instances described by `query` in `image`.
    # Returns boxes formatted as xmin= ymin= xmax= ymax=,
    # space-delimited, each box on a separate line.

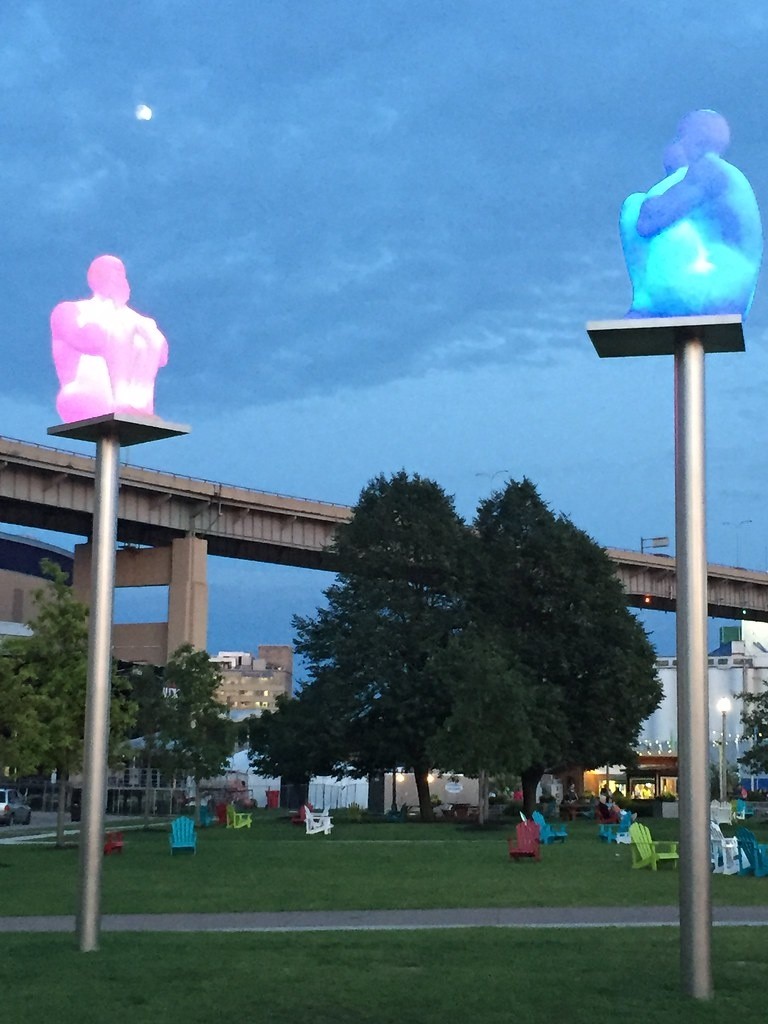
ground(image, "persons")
xmin=539 ymin=782 xmax=622 ymax=820
xmin=619 ymin=110 xmax=761 ymax=318
xmin=733 ymin=783 xmax=747 ymax=800
xmin=51 ymin=255 xmax=170 ymax=422
xmin=68 ymin=764 xmax=84 ymax=821
xmin=488 ymin=784 xmax=524 ymax=800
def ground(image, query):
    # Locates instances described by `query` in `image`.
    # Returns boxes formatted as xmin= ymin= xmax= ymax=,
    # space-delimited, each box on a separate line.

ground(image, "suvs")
xmin=0 ymin=786 xmax=32 ymax=827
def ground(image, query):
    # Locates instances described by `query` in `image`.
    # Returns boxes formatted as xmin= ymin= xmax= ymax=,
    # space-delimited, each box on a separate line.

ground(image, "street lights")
xmin=716 ymin=696 xmax=732 ymax=804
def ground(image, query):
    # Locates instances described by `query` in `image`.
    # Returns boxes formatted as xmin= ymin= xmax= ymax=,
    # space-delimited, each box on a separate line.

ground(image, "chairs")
xmin=226 ymin=805 xmax=253 ymax=829
xmin=168 ymin=816 xmax=198 ymax=856
xmin=730 ymin=800 xmax=737 ymax=822
xmin=710 ymin=799 xmax=720 ymax=822
xmin=710 ymin=821 xmax=751 ymax=875
xmin=736 ymin=826 xmax=768 ymax=877
xmin=304 ymin=806 xmax=333 ymax=834
xmin=597 ymin=811 xmax=632 ymax=843
xmin=104 ymin=830 xmax=123 ymax=854
xmin=216 ymin=803 xmax=227 ymax=823
xmin=508 ymin=820 xmax=541 ymax=862
xmin=532 ymin=811 xmax=567 ymax=844
xmin=199 ymin=806 xmax=217 ymax=827
xmin=737 ymin=799 xmax=745 ymax=819
xmin=293 ymin=803 xmax=313 ymax=826
xmin=309 ymin=805 xmax=331 ymax=832
xmin=628 ymin=821 xmax=679 ymax=871
xmin=718 ymin=801 xmax=733 ymax=826
xmin=597 ymin=809 xmax=620 ymax=824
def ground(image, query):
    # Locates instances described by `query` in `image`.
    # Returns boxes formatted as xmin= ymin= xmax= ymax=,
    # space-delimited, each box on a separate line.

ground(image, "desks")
xmin=448 ymin=802 xmax=471 ymax=816
xmin=560 ymin=803 xmax=596 ymax=822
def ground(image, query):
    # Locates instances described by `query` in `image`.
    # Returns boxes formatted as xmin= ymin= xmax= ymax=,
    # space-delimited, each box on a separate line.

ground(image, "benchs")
xmin=442 ymin=808 xmax=455 ymax=816
xmin=468 ymin=805 xmax=479 ymax=815
